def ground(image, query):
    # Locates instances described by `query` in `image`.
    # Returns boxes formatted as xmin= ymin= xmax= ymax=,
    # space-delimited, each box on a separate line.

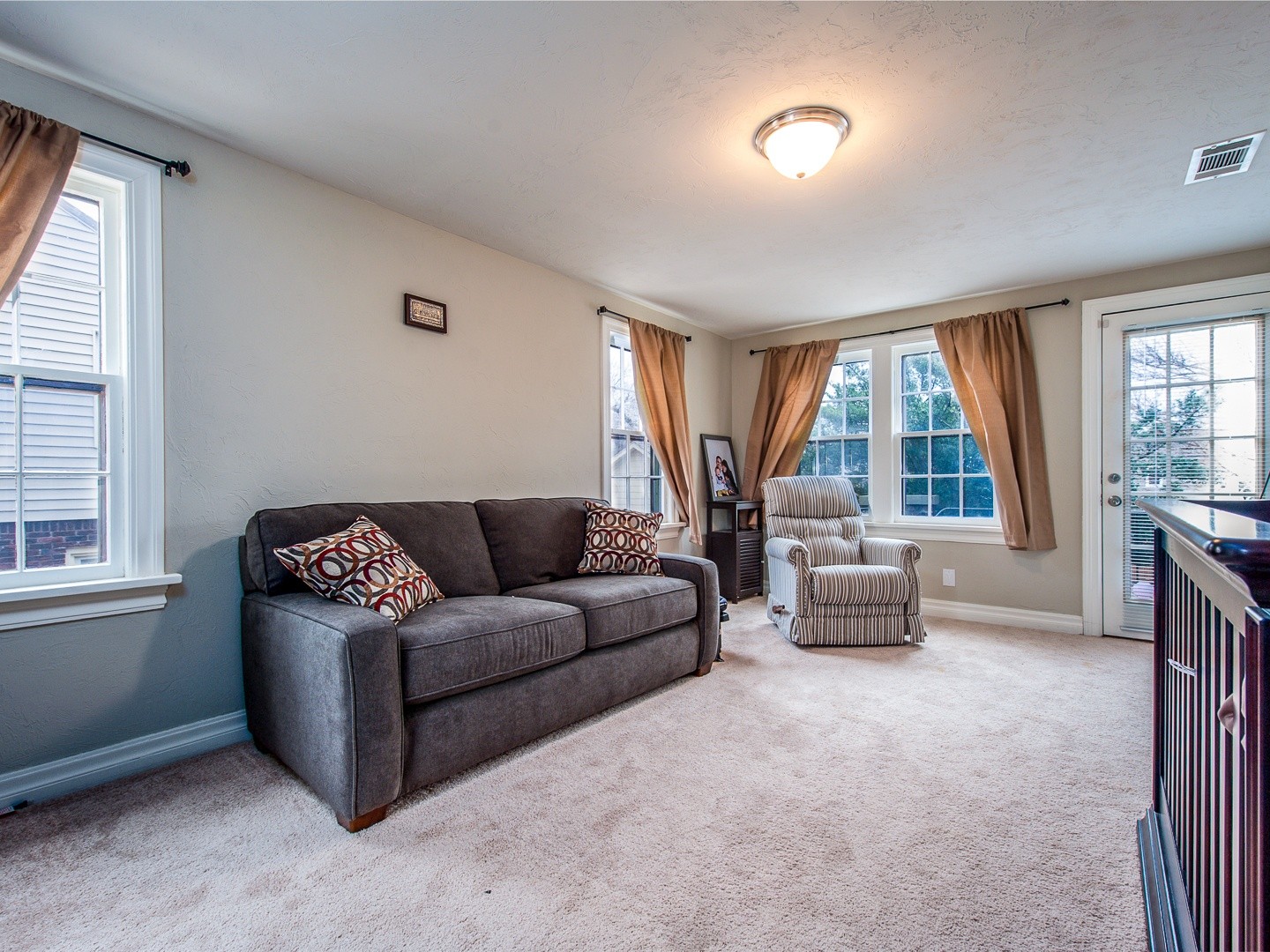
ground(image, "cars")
xmin=1160 ymin=476 xmax=1184 ymax=491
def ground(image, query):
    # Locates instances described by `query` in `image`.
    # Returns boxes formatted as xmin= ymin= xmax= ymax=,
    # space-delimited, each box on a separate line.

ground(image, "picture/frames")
xmin=700 ymin=433 xmax=744 ymax=503
xmin=403 ymin=292 xmax=448 ymax=334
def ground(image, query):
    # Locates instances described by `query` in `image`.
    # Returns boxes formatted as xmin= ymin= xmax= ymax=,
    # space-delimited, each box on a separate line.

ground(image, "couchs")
xmin=760 ymin=474 xmax=929 ymax=648
xmin=237 ymin=495 xmax=719 ymax=835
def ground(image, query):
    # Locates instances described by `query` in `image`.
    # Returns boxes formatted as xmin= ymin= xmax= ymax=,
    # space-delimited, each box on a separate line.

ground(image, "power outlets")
xmin=942 ymin=568 xmax=956 ymax=587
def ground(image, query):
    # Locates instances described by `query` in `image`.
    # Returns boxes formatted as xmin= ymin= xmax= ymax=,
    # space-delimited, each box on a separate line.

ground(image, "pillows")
xmin=576 ymin=500 xmax=665 ymax=577
xmin=271 ymin=514 xmax=448 ymax=628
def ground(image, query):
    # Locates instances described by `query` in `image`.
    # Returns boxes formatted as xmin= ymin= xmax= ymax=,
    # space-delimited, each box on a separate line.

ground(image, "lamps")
xmin=753 ymin=106 xmax=851 ymax=180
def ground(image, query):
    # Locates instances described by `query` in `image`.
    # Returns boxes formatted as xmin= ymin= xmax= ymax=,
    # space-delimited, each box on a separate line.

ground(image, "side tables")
xmin=706 ymin=497 xmax=765 ymax=605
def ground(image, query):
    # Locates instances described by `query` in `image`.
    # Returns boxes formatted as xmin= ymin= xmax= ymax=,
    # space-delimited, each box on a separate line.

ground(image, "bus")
xmin=1146 ymin=466 xmax=1162 ymax=484
